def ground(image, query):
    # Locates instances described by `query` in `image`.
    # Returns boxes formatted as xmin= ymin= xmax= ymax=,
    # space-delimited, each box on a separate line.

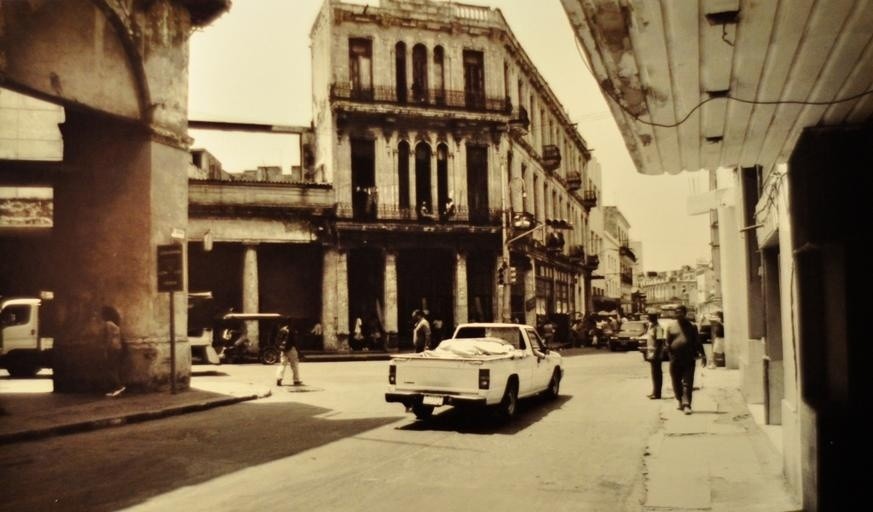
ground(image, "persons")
xmin=712 ymin=310 xmax=725 ymax=358
xmin=665 ymin=303 xmax=708 ymax=415
xmin=353 ymin=316 xmax=364 ymax=348
xmin=411 ymin=308 xmax=432 ymax=353
xmin=571 ymin=315 xmax=635 ymax=348
xmin=275 ymin=314 xmax=302 ymax=386
xmin=214 ymin=304 xmax=236 ymax=356
xmin=311 ymin=319 xmax=323 ymax=351
xmin=88 ymin=304 xmax=126 ymax=397
xmin=639 ymin=306 xmax=664 ymax=398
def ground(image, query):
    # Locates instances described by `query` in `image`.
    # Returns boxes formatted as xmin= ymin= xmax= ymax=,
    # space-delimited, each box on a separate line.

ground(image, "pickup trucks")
xmin=385 ymin=323 xmax=565 ymax=421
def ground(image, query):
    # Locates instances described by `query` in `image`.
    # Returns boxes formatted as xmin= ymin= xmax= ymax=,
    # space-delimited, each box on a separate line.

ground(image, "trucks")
xmin=1 ymin=292 xmax=220 ymax=379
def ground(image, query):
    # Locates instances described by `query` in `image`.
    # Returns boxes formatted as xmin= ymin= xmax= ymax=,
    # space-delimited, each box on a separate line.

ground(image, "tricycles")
xmin=215 ymin=310 xmax=288 ymax=363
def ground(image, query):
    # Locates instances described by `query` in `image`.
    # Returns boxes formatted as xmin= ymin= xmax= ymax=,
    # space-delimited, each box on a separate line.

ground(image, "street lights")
xmin=501 ymin=175 xmax=527 ymax=323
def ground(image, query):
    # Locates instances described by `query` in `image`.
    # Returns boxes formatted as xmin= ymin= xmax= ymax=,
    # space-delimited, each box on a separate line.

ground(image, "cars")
xmin=589 ymin=321 xmax=678 ymax=361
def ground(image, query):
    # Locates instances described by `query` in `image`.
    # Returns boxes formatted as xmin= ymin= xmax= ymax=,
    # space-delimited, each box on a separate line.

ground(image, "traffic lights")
xmin=544 ymin=218 xmax=570 ymax=226
xmin=496 ymin=267 xmax=517 ymax=284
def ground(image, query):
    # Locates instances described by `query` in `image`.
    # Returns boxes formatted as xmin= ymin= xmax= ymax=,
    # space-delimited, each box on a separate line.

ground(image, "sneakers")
xmin=105 ymin=386 xmax=127 ymax=398
xmin=646 ymin=392 xmax=693 ymax=416
xmin=275 ymin=377 xmax=304 ymax=388
